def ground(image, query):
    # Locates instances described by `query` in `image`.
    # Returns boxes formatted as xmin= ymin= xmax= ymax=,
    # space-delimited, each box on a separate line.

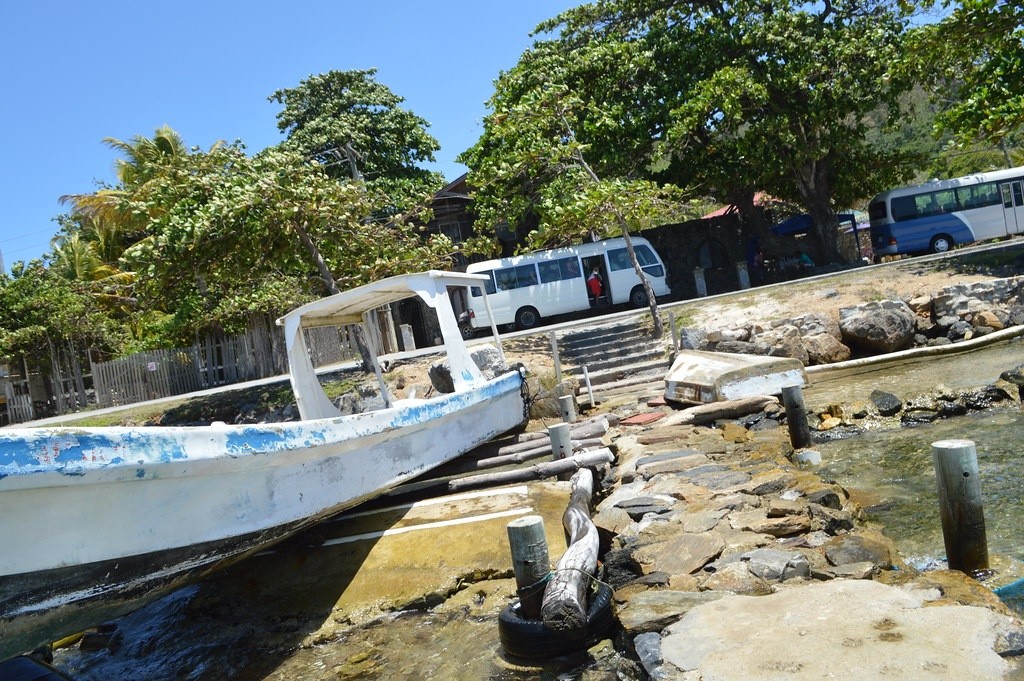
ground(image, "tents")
xmin=750 ymin=214 xmax=862 ymax=273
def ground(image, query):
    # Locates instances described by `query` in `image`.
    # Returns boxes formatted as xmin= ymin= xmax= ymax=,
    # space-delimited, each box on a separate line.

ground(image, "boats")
xmin=0 ymin=269 xmax=530 ymax=665
xmin=663 ymin=345 xmax=814 ymax=407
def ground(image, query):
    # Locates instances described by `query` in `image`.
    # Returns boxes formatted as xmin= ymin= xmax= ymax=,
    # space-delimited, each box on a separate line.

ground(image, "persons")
xmin=795 ymin=251 xmax=816 ymax=277
xmin=586 ymin=266 xmax=603 ymax=305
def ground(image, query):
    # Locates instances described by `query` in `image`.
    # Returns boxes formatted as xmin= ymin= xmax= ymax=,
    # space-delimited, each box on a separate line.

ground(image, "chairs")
xmin=912 ymin=193 xmax=1004 ymax=219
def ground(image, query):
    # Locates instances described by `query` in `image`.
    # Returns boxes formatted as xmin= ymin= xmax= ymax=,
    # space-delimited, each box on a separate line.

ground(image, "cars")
xmin=457 ymin=309 xmax=517 ymax=340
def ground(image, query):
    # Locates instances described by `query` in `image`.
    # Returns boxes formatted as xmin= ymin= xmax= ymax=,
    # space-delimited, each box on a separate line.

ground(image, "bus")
xmin=868 ymin=167 xmax=1024 ymax=256
xmin=465 ymin=237 xmax=671 ymax=332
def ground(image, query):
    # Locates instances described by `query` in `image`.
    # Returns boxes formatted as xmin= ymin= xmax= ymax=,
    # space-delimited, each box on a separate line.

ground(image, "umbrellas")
xmin=844 ymin=224 xmax=871 ymax=234
xmin=834 ymin=208 xmax=869 ymax=226
